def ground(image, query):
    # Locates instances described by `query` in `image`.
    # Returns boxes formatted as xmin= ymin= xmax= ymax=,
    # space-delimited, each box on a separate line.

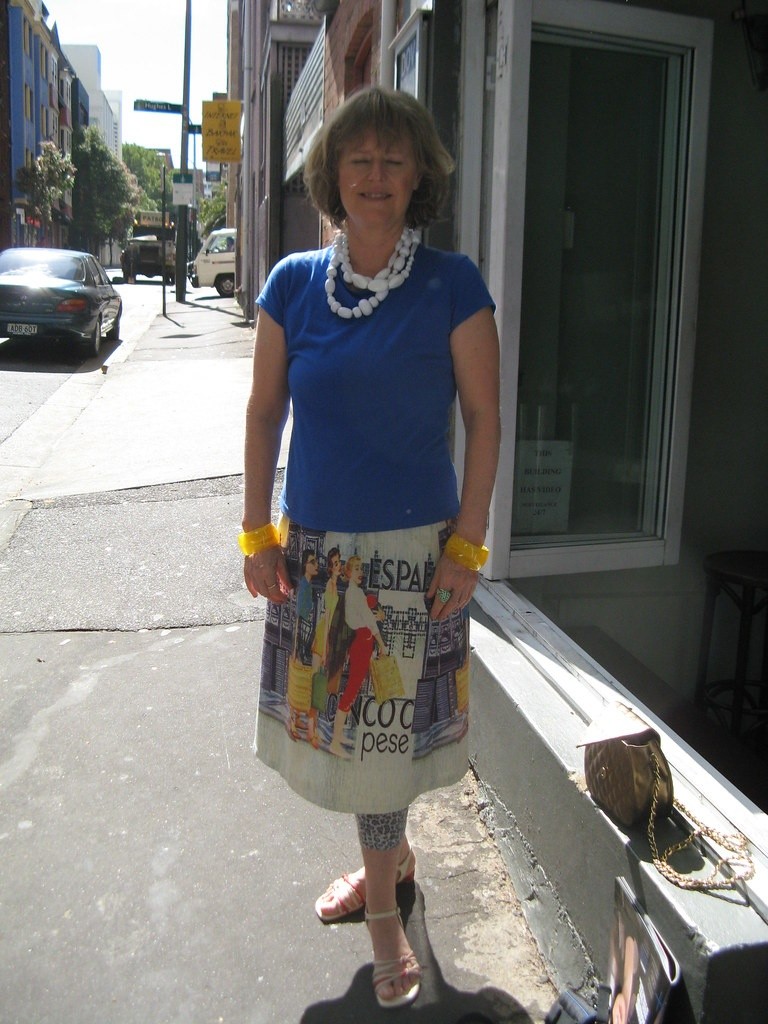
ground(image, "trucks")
xmin=120 ymin=211 xmax=177 ymax=285
xmin=186 ymin=228 xmax=236 ymax=299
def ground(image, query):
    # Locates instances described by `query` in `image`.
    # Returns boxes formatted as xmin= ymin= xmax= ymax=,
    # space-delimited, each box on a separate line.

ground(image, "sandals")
xmin=364 ymin=906 xmax=421 ymax=1008
xmin=314 ymin=844 xmax=414 ymax=922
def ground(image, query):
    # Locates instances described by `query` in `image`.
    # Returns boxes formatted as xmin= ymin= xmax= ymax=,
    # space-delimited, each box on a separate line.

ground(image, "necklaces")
xmin=326 ymin=224 xmax=421 ymax=318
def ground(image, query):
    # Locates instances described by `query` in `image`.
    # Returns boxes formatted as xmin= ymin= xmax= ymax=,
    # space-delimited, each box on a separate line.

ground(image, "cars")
xmin=0 ymin=246 xmax=123 ymax=358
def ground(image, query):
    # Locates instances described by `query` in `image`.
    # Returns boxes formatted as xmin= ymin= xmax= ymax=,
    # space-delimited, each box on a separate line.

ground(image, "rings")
xmin=268 ymin=580 xmax=279 ymax=589
xmin=436 ymin=588 xmax=451 ymax=603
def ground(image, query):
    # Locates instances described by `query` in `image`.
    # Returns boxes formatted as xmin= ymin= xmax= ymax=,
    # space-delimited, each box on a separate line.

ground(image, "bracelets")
xmin=237 ymin=522 xmax=280 ymax=555
xmin=444 ymin=533 xmax=491 ymax=571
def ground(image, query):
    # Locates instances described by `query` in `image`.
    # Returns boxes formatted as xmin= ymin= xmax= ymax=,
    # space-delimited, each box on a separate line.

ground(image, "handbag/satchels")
xmin=545 ymin=984 xmax=611 ymax=1024
xmin=576 ymin=728 xmax=754 ymax=889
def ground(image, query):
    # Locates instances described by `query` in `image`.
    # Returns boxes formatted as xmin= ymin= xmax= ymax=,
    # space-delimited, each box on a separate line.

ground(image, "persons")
xmin=611 ymin=880 xmax=640 ymax=1024
xmin=242 ymin=85 xmax=502 ymax=1008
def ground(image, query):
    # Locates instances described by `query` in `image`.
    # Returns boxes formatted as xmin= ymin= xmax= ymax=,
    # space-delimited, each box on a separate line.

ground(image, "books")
xmin=607 ymin=876 xmax=681 ymax=1024
